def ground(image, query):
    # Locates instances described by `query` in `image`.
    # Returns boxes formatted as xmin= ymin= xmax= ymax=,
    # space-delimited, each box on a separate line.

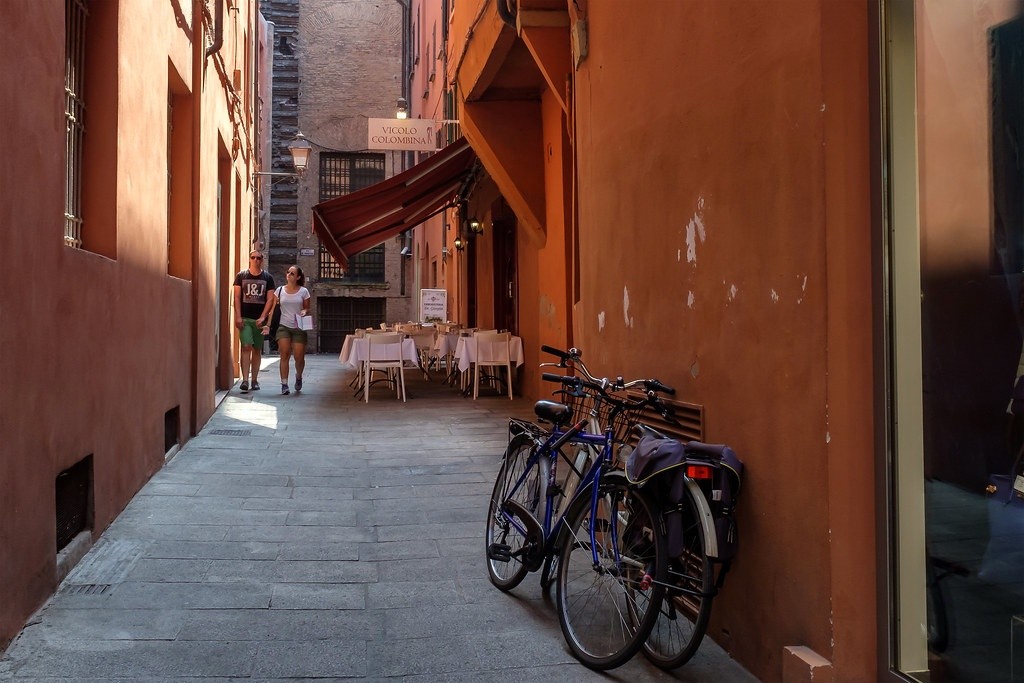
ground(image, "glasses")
xmin=250 ymin=256 xmax=261 ymax=260
xmin=287 ymin=271 xmax=295 ymax=275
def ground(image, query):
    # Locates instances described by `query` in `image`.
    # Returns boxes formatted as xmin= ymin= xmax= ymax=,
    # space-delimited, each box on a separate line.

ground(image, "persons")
xmin=261 ymin=265 xmax=311 ymax=395
xmin=233 ymin=250 xmax=275 ymax=392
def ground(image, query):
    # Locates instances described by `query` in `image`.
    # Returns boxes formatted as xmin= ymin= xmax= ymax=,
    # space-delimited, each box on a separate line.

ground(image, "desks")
xmin=341 ymin=328 xmax=524 ymax=399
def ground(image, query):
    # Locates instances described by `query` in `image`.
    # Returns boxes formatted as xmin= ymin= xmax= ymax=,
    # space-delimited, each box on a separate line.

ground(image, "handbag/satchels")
xmin=269 ymin=286 xmax=283 ymax=336
xmin=623 ymin=436 xmax=685 ymax=560
xmin=686 ymin=441 xmax=744 ymax=562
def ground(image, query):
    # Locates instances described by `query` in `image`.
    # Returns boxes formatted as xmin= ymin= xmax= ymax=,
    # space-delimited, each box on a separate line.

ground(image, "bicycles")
xmin=485 ymin=343 xmax=739 ymax=672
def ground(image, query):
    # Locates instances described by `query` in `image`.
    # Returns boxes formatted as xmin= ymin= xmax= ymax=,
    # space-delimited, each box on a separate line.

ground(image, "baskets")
xmin=562 ymin=384 xmax=645 ymax=446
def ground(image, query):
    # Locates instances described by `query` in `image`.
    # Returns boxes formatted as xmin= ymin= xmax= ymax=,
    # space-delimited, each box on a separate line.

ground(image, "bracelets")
xmin=266 ymin=325 xmax=270 ymax=327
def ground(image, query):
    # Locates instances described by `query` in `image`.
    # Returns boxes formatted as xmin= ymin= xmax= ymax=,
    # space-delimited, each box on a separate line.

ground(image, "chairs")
xmin=355 ymin=318 xmax=512 ymax=403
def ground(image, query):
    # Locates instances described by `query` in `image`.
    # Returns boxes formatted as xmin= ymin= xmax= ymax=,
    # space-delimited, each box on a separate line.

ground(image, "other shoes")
xmin=240 ymin=381 xmax=248 ymax=390
xmin=252 ymin=384 xmax=260 ymax=390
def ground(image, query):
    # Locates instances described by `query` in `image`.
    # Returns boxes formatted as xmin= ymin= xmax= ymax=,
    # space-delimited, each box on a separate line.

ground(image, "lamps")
xmin=471 ymin=218 xmax=483 ymax=235
xmin=396 ymin=96 xmax=409 ymax=119
xmin=454 ymin=237 xmax=465 ymax=252
xmin=251 ymin=132 xmax=315 ymax=203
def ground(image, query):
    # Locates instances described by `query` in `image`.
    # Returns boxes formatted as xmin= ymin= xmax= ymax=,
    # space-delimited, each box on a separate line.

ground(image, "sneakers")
xmin=295 ymin=374 xmax=302 ymax=390
xmin=282 ymin=384 xmax=290 ymax=395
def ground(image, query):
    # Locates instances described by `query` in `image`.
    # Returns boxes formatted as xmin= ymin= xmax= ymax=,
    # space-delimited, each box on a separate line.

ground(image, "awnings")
xmin=310 ymin=135 xmax=477 ymax=268
